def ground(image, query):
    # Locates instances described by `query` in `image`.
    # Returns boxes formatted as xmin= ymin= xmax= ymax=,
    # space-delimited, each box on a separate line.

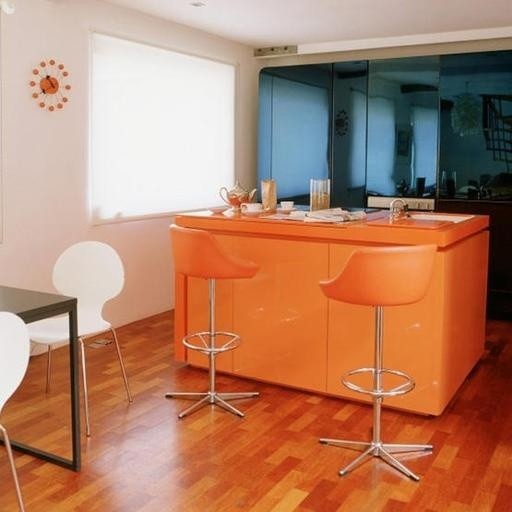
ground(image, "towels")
xmin=306 ymin=207 xmax=366 ymax=221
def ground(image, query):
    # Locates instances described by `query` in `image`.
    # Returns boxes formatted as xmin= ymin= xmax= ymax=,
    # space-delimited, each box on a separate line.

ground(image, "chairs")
xmin=319 ymin=244 xmax=439 ymax=480
xmin=0 ymin=312 xmax=31 ymax=512
xmin=165 ymin=224 xmax=258 ymax=419
xmin=25 ymin=241 xmax=133 ymax=436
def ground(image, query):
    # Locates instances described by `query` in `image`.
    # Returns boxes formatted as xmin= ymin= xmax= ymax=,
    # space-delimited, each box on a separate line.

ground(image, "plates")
xmin=208 ymin=206 xmax=232 ymax=214
xmin=276 ymin=208 xmax=297 ymax=214
xmin=240 ymin=209 xmax=266 ymax=216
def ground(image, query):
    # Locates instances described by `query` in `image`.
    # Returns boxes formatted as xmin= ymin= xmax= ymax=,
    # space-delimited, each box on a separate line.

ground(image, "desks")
xmin=0 ymin=286 xmax=81 ymax=472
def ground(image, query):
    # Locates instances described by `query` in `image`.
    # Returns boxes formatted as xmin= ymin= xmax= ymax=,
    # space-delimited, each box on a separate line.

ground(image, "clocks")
xmin=30 ymin=60 xmax=74 ymax=109
xmin=336 ymin=108 xmax=350 ymax=135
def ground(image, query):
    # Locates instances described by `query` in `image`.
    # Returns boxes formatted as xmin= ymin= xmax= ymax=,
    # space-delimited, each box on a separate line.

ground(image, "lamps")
xmin=451 ymin=81 xmax=483 ymax=137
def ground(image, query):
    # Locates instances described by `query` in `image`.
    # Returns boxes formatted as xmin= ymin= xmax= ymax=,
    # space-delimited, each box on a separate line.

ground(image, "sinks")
xmin=403 ymin=213 xmax=474 ymax=224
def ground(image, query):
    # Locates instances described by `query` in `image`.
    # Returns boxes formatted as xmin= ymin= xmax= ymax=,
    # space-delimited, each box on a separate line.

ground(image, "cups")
xmin=310 ymin=178 xmax=331 ymax=212
xmin=261 ymin=178 xmax=277 ymax=212
xmin=240 ymin=202 xmax=263 ymax=211
xmin=280 ymin=202 xmax=294 ymax=209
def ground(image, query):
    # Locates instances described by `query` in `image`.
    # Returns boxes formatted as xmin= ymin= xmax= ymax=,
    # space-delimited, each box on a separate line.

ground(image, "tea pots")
xmin=219 ymin=179 xmax=258 ymax=211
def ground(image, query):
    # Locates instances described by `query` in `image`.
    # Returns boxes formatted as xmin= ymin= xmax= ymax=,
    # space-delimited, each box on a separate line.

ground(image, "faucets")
xmin=390 ymin=198 xmax=408 ymax=215
